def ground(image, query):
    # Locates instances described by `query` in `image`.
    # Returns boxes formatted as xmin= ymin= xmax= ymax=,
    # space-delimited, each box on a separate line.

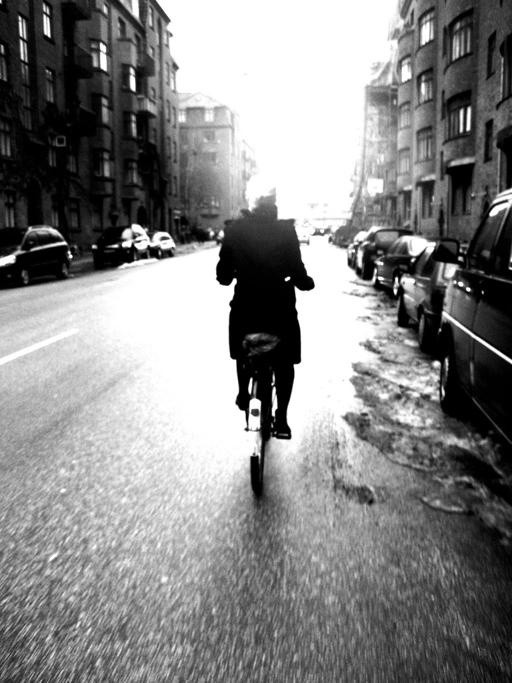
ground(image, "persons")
xmin=215 ymin=177 xmax=316 ymax=434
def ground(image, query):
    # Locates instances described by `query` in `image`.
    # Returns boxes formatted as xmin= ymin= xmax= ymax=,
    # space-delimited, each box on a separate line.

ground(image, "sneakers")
xmin=235 ymin=390 xmax=250 ymax=412
xmin=273 ymin=419 xmax=291 ymax=441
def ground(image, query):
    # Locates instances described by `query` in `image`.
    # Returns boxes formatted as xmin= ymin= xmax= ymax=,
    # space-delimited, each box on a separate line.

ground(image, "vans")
xmin=436 ymin=186 xmax=511 ymax=449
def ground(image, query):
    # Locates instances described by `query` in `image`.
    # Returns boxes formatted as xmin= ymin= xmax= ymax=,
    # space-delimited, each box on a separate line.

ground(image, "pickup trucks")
xmin=396 ymin=246 xmax=463 ymax=353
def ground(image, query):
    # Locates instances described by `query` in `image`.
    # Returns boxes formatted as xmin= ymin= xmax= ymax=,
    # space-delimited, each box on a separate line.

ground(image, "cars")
xmin=346 ymin=231 xmax=365 ymax=268
xmin=297 ymin=231 xmax=311 ymax=245
xmin=372 ymin=236 xmax=437 ymax=297
xmin=196 ymin=226 xmax=226 ymax=244
xmin=90 ymin=224 xmax=151 ymax=270
xmin=1 ymin=224 xmax=73 ymax=287
xmin=356 ymin=226 xmax=412 ymax=281
xmin=147 ymin=229 xmax=176 ymax=258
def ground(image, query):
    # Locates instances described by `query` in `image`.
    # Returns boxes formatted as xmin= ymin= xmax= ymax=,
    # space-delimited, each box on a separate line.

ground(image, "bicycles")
xmin=215 ymin=269 xmax=311 ymax=496
